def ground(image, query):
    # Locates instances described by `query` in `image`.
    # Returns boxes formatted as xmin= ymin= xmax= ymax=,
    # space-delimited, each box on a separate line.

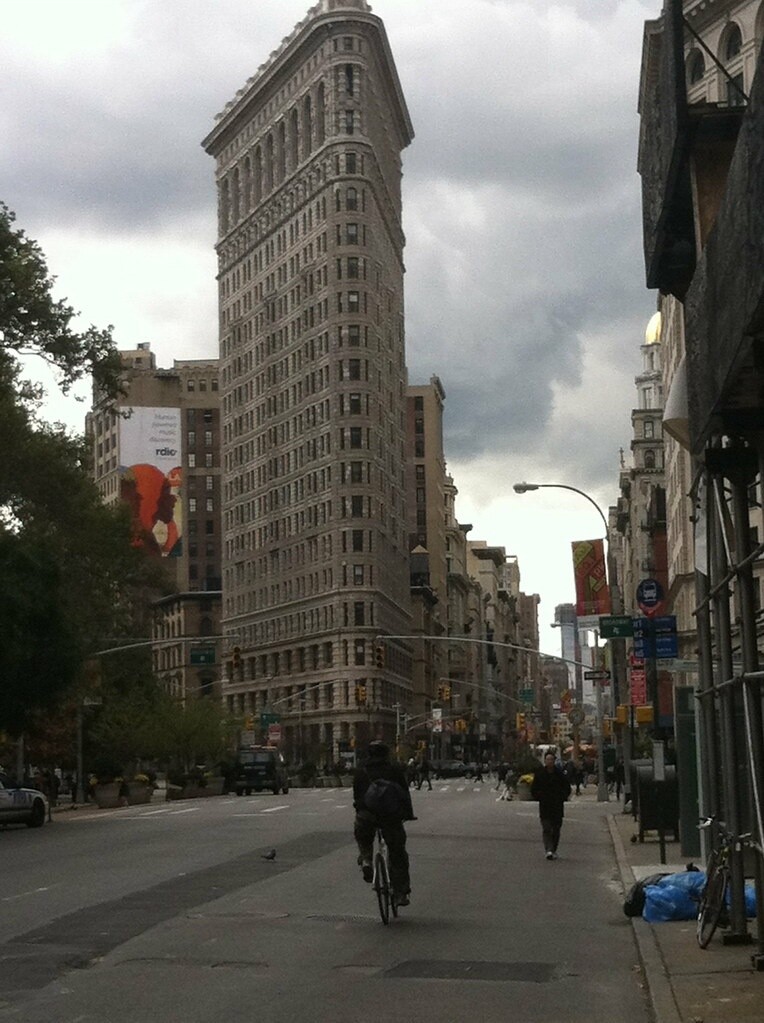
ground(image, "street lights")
xmin=512 ymin=482 xmax=609 ymax=541
xmin=550 ymin=623 xmax=609 ymax=802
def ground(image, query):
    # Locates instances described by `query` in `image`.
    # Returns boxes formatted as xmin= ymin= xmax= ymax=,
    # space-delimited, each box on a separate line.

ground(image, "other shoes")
xmin=545 ymin=852 xmax=553 ymax=859
xmin=553 ymin=854 xmax=556 ymax=860
xmin=362 ymin=860 xmax=374 ymax=883
xmin=397 ymin=894 xmax=409 ymax=905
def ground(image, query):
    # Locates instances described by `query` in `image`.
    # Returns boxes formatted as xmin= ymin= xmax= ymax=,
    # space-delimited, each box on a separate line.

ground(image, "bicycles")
xmin=371 ymin=817 xmax=419 ymax=926
xmin=696 ymin=813 xmax=764 ymax=948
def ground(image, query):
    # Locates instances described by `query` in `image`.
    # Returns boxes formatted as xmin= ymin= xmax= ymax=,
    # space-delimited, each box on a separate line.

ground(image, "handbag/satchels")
xmin=364 ymin=779 xmax=408 ymax=827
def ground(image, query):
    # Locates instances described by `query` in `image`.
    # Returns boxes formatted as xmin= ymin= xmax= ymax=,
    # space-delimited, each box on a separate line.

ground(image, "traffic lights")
xmin=456 ymin=719 xmax=466 ymax=730
xmin=584 ymin=670 xmax=611 ymax=680
xmin=396 ymin=734 xmax=400 ymax=744
xmin=233 ymin=645 xmax=242 ymax=670
xmin=616 ymin=706 xmax=626 ymax=724
xmin=517 ymin=712 xmax=525 ymax=729
xmin=358 ymin=686 xmax=367 ymax=701
xmin=436 ymin=685 xmax=450 ymax=701
xmin=375 ymin=646 xmax=385 ymax=669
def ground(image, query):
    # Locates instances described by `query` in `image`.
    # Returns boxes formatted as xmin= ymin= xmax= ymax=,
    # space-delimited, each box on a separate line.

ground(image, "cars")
xmin=440 ymin=760 xmax=511 ymax=777
xmin=0 ymin=771 xmax=50 ymax=829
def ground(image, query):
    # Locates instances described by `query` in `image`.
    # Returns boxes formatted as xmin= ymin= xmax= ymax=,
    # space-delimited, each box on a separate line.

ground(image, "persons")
xmin=474 ymin=762 xmax=484 ymax=783
xmin=500 ymin=770 xmax=514 ymax=800
xmin=495 ymin=760 xmax=507 ymax=791
xmin=353 ymin=745 xmax=413 ymax=905
xmin=408 ymin=757 xmax=417 ymax=786
xmin=606 ymin=759 xmax=625 ymax=793
xmin=415 ymin=754 xmax=432 ymax=790
xmin=89 ymin=774 xmax=98 ymax=785
xmin=47 ymin=769 xmax=61 ymax=800
xmin=575 ymin=769 xmax=585 ymax=795
xmin=531 ymin=752 xmax=572 ymax=860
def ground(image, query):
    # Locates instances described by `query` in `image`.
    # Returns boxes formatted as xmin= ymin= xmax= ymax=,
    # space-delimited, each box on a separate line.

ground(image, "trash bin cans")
xmin=636 ymin=766 xmax=680 ymax=865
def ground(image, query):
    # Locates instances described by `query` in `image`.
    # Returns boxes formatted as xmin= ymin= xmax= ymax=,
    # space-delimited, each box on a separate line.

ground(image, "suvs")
xmin=233 ymin=745 xmax=292 ymax=795
